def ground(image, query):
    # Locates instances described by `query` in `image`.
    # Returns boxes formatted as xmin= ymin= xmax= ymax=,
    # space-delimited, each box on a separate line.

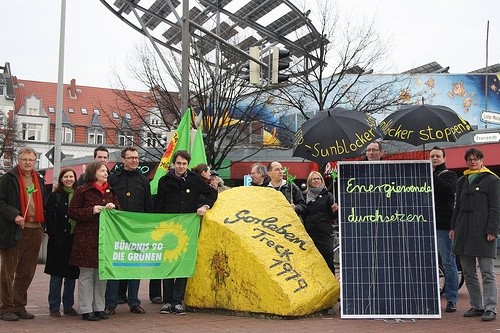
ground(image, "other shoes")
xmin=15 ymin=310 xmax=35 ymax=318
xmin=105 ymin=307 xmax=115 ymax=314
xmin=130 ymin=305 xmax=146 ymax=314
xmin=50 ymin=309 xmax=61 ymax=316
xmin=83 ymin=313 xmax=99 ymax=320
xmin=153 ymin=299 xmax=163 ymax=304
xmin=0 ymin=312 xmax=18 ymax=320
xmin=94 ymin=311 xmax=108 ymax=319
xmin=64 ymin=308 xmax=79 ymax=316
xmin=124 ymin=297 xmax=128 ymax=302
xmin=117 ymin=300 xmax=124 ymax=304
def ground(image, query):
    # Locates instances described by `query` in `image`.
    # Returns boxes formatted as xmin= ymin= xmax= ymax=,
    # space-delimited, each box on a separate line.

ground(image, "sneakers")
xmin=464 ymin=308 xmax=482 ymax=317
xmin=446 ymin=301 xmax=456 ymax=311
xmin=481 ymin=310 xmax=495 ymax=320
xmin=160 ymin=303 xmax=173 ymax=313
xmin=172 ymin=304 xmax=186 ymax=314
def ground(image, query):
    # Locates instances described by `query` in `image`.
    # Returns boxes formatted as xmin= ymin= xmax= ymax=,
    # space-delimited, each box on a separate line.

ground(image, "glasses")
xmin=125 ymin=157 xmax=139 ymax=160
xmin=467 ymin=158 xmax=480 ymax=162
xmin=365 ymin=148 xmax=381 ymax=153
xmin=311 ymin=177 xmax=322 ymax=181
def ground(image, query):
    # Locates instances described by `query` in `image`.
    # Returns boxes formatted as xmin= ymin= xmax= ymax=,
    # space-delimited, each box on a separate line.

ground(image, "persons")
xmin=430 ymin=146 xmax=460 ymax=313
xmin=363 ymin=141 xmax=385 ymax=162
xmin=69 ymin=145 xmax=151 ymax=322
xmin=190 ymin=163 xmax=231 ymax=193
xmin=0 ymin=147 xmax=48 ymax=320
xmin=150 ymin=149 xmax=218 ymax=316
xmin=44 ymin=167 xmax=80 ymax=319
xmin=448 ymin=148 xmax=500 ymax=321
xmin=250 ymin=162 xmax=270 ymax=187
xmin=300 ymin=170 xmax=338 ymax=278
xmin=266 ymin=160 xmax=306 ymax=216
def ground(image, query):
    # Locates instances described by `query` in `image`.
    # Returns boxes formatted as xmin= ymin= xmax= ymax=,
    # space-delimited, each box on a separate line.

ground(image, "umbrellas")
xmin=292 ymin=106 xmax=386 ymax=169
xmin=375 ymin=96 xmax=474 ymax=161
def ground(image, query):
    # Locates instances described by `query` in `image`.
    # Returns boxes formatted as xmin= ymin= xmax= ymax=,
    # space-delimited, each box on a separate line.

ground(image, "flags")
xmin=150 ymin=108 xmax=190 ymax=196
xmin=189 ymin=126 xmax=208 ymax=172
